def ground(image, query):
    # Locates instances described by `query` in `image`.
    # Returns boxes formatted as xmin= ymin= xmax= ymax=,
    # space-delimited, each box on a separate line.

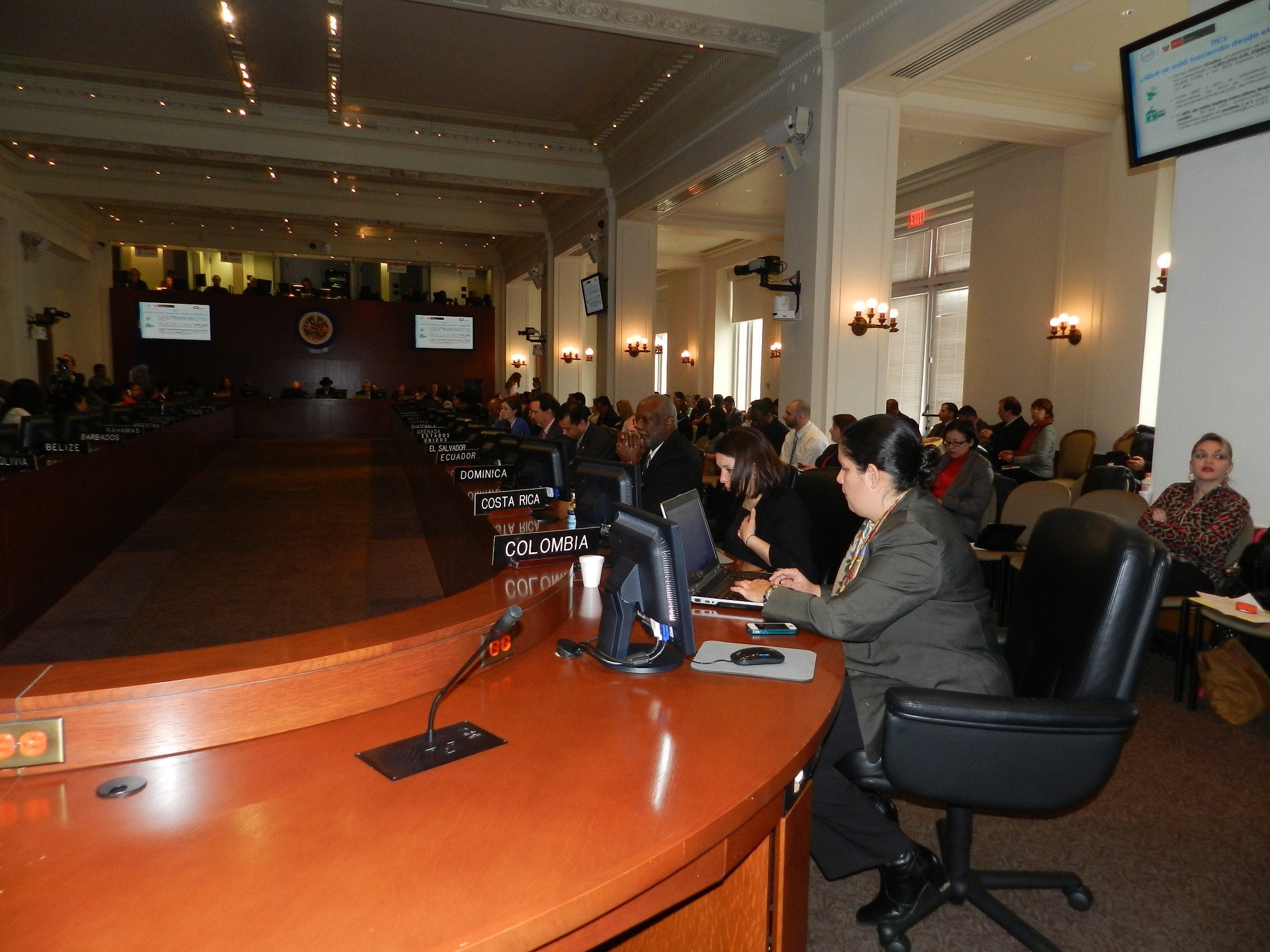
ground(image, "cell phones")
xmin=746 ymin=622 xmax=798 ymax=635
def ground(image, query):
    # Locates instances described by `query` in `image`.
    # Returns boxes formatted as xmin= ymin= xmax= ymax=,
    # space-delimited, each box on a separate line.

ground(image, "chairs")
xmin=1163 ymin=526 xmax=1270 ymax=713
xmin=874 ymin=508 xmax=1169 ymax=952
xmin=968 ymin=429 xmax=1138 ymax=630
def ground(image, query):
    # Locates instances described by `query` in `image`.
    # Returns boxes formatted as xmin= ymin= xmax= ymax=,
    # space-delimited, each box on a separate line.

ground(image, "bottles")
xmin=568 ymin=491 xmax=577 ymax=529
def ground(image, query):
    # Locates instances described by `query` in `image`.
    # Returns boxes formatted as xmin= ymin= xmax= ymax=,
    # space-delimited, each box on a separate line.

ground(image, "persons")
xmin=731 ymin=412 xmax=1019 ymax=925
xmin=469 ymin=371 xmax=1056 ymax=513
xmin=1127 ymin=456 xmax=1151 ymax=475
xmin=922 ymin=418 xmax=992 ymax=543
xmin=219 ymin=378 xmax=470 ymax=410
xmin=1136 ymin=434 xmax=1250 ymax=593
xmin=0 ymin=353 xmax=171 ymax=430
xmin=715 ymin=426 xmax=819 ymax=584
xmin=129 ymin=268 xmax=448 ymax=304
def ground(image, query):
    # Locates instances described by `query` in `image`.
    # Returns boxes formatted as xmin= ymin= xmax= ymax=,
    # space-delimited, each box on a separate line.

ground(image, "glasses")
xmin=942 ymin=439 xmax=968 ymax=447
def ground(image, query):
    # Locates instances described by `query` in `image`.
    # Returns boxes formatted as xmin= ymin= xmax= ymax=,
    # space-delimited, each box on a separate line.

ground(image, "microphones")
xmin=355 ymin=605 xmax=524 ymax=780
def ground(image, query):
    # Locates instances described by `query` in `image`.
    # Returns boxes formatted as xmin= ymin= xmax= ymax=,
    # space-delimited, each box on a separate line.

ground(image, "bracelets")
xmin=763 ymin=584 xmax=781 ymax=607
xmin=746 ymin=534 xmax=756 ymax=546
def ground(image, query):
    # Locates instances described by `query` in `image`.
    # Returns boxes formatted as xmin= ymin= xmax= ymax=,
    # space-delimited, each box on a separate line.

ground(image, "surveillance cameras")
xmin=734 ymin=255 xmax=781 ymax=285
xmin=27 ymin=307 xmax=70 ymax=324
xmin=518 ymin=327 xmax=539 ymax=339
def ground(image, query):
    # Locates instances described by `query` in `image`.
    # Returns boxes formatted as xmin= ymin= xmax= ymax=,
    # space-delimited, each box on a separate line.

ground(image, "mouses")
xmin=731 ymin=647 xmax=785 ymax=666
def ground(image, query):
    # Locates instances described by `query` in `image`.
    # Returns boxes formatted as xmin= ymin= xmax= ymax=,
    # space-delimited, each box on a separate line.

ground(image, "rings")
xmin=1161 ymin=520 xmax=1164 ymax=523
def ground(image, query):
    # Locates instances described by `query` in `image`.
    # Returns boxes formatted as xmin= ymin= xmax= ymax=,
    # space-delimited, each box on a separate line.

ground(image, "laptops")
xmin=660 ymin=489 xmax=781 ymax=612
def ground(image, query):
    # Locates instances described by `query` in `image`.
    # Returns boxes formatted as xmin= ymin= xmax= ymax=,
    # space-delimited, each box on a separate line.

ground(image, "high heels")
xmin=856 ymin=840 xmax=951 ymax=926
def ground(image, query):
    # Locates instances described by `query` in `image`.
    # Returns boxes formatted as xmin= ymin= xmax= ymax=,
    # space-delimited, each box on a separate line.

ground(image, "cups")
xmin=580 ymin=553 xmax=604 ymax=587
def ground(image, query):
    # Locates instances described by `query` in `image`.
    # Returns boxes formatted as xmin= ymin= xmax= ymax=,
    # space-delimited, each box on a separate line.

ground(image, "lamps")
xmin=654 ymin=338 xmax=663 ymax=354
xmin=560 ymin=347 xmax=581 ymax=363
xmin=681 ymin=350 xmax=694 ymax=367
xmin=770 ymin=342 xmax=782 ymax=358
xmin=1046 ymin=313 xmax=1082 ymax=345
xmin=624 ymin=335 xmax=651 ymax=357
xmin=510 ymin=354 xmax=527 ymax=368
xmin=848 ymin=298 xmax=899 ymax=336
xmin=585 ymin=348 xmax=594 ymax=361
xmin=1152 ymin=251 xmax=1171 ymax=294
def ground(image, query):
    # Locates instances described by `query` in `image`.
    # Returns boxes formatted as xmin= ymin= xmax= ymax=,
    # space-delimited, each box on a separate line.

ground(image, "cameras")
xmin=330 ymin=389 xmax=347 ymax=399
xmin=56 ymin=356 xmax=69 ymax=372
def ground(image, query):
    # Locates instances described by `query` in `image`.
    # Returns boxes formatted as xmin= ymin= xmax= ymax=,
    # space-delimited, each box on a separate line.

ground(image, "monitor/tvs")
xmin=476 ymin=426 xmax=513 ymax=465
xmin=495 ymin=433 xmax=540 ymax=490
xmin=572 ymin=455 xmax=642 ymax=548
xmin=515 ymin=435 xmax=572 ymax=523
xmin=256 ymin=279 xmax=331 ymax=297
xmin=239 ymin=386 xmax=259 ymax=398
xmin=0 ymin=422 xmax=19 ymax=454
xmin=370 ymin=389 xmax=388 ymax=399
xmin=595 ymin=500 xmax=696 ymax=674
xmin=412 ymin=313 xmax=476 ymax=352
xmin=108 ymin=395 xmax=206 ymax=424
xmin=137 ymin=299 xmax=214 ymax=344
xmin=1119 ymin=0 xmax=1270 ymax=170
xmin=406 ymin=399 xmax=491 ymax=459
xmin=61 ymin=410 xmax=105 ymax=443
xmin=20 ymin=415 xmax=54 ymax=459
xmin=581 ymin=273 xmax=607 ymax=316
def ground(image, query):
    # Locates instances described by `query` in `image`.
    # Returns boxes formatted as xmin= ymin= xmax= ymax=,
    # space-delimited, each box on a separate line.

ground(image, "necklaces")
xmin=748 ymin=495 xmax=762 ymax=507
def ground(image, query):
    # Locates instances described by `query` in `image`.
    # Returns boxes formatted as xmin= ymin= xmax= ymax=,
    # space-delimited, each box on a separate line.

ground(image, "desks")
xmin=0 ymin=392 xmax=846 ymax=952
